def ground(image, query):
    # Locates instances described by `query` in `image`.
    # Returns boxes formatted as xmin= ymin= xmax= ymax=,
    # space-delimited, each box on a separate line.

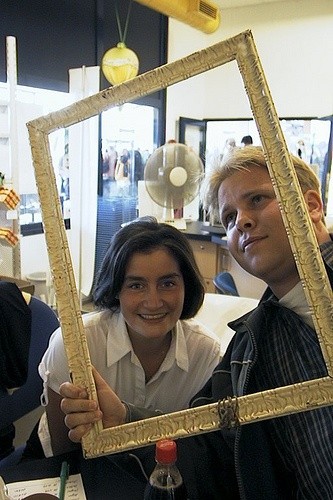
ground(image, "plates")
xmin=21 ymin=493 xmax=61 ymax=500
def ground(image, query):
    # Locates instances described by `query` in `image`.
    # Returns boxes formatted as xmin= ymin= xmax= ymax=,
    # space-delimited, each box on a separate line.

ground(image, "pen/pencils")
xmin=58 ymin=460 xmax=70 ymax=500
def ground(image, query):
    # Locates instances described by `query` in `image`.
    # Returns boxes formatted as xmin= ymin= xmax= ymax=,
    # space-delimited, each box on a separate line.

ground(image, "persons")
xmin=207 ymin=149 xmax=333 ymax=500
xmin=39 ymin=220 xmax=225 ymax=431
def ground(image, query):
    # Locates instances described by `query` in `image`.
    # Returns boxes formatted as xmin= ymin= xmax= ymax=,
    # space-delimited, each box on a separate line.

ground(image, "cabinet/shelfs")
xmin=179 ymin=222 xmax=231 ymax=294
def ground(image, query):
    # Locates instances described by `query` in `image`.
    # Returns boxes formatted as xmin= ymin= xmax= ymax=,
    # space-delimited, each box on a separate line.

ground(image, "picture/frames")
xmin=26 ymin=30 xmax=333 ymax=459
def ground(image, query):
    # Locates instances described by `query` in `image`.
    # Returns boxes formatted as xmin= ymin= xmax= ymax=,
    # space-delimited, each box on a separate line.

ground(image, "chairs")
xmin=212 ymin=272 xmax=239 ymax=297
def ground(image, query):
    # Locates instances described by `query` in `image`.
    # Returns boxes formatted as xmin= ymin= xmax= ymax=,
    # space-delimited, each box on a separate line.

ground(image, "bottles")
xmin=144 ymin=439 xmax=188 ymax=500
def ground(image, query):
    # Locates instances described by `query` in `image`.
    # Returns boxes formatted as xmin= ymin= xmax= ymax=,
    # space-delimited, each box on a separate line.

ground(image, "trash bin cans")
xmin=27 ymin=271 xmax=55 ymax=312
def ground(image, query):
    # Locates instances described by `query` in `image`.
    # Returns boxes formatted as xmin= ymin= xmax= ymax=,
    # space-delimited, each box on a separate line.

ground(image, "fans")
xmin=144 ymin=143 xmax=205 ymax=227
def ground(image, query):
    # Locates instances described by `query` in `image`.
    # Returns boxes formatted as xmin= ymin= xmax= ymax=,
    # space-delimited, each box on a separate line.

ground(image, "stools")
xmin=27 ymin=272 xmax=49 ymax=304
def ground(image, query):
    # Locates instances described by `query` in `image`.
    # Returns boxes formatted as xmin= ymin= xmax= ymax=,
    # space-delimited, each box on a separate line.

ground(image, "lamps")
xmin=102 ymin=1 xmax=139 ymax=86
